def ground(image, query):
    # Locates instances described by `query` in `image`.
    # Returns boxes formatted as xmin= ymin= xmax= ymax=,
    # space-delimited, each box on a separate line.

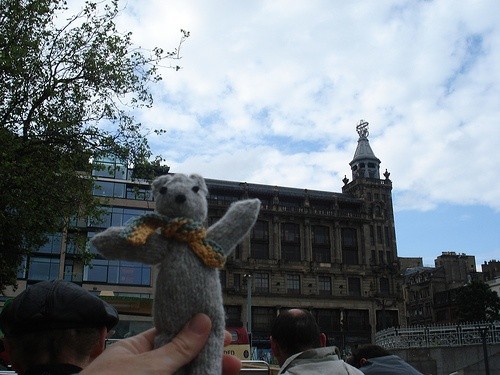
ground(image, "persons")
xmin=358 ymin=342 xmax=426 ymax=375
xmin=268 ymin=306 xmax=366 ymax=375
xmin=1 ymin=278 xmax=213 ymax=374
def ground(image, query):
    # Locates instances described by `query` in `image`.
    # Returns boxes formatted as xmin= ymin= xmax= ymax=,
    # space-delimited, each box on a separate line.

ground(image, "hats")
xmin=0 ymin=279 xmax=119 ymax=331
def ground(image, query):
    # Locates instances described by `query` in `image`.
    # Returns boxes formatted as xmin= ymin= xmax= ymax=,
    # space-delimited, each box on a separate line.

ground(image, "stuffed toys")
xmin=94 ymin=172 xmax=262 ymax=375
